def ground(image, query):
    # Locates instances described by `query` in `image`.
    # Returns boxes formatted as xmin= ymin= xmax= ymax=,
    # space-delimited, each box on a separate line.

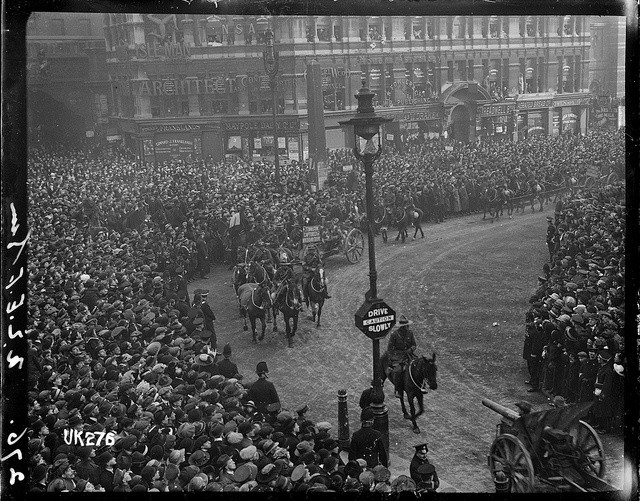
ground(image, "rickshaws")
xmin=479 ymin=389 xmax=624 ymax=495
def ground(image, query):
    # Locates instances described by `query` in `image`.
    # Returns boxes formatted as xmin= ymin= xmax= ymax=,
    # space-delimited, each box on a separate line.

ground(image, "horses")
xmin=232 ymin=262 xmax=247 ymax=304
xmin=391 ymin=207 xmax=426 ymax=244
xmin=267 ymin=284 xmax=303 ymax=350
xmin=372 ymin=198 xmax=409 ymax=242
xmin=294 ymin=261 xmax=327 ymax=329
xmin=378 ymin=351 xmax=438 ymax=435
xmin=237 ymin=282 xmax=274 ymax=346
xmin=245 ymin=260 xmax=273 ymax=286
xmin=231 ymin=261 xmax=247 ymax=289
xmin=477 ymin=169 xmax=579 ymax=222
xmin=250 ymin=247 xmax=293 ymax=265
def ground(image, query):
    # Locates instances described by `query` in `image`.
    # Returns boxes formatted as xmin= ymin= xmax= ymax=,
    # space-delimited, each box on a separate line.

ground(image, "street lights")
xmin=350 ymin=80 xmax=392 ymax=469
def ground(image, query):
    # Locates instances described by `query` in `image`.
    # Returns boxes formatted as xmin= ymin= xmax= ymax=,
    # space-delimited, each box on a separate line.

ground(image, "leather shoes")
xmin=527 ymin=387 xmax=537 ymax=391
xmin=525 ymin=380 xmax=530 ymax=383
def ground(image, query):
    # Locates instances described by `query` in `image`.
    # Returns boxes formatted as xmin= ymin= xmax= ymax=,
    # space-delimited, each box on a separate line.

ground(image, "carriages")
xmin=480 ymin=161 xmax=620 ymax=223
xmin=232 ymin=211 xmax=364 ymax=349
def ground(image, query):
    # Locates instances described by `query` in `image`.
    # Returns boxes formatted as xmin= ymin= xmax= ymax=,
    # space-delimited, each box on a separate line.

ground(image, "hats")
xmin=140 ymin=299 xmax=150 ymax=308
xmin=156 ymin=375 xmax=172 ymax=387
xmin=167 ymin=448 xmax=185 ymax=465
xmin=171 ymin=346 xmax=180 ymax=354
xmin=188 ymin=409 xmax=202 ymax=422
xmin=273 ymin=475 xmax=291 ymax=492
xmin=198 ymin=230 xmax=204 ymax=234
xmin=572 ymin=315 xmax=584 ymax=323
xmin=324 ymin=439 xmax=339 ymax=451
xmin=154 ymin=293 xmax=163 ymax=299
xmin=84 ymin=279 xmax=94 ymax=287
xmin=275 ymin=222 xmax=284 ymax=227
xmin=147 ymin=342 xmax=161 ymax=355
xmin=299 ymin=453 xmax=315 ymax=463
xmin=48 ymin=373 xmax=61 ymax=383
xmin=223 ymin=384 xmax=238 ymax=396
xmin=58 ymin=308 xmax=67 ymax=319
xmin=79 ymin=366 xmax=90 ymax=378
xmin=564 ymin=326 xmax=579 ymax=342
xmin=160 ymin=300 xmax=170 ymax=308
xmin=68 ymin=408 xmax=81 ymax=417
xmin=245 ymin=400 xmax=257 ymax=409
xmin=265 ymin=227 xmax=274 ymax=234
xmin=413 ymin=443 xmax=428 ymax=454
xmin=153 ymin=282 xmax=162 ymax=288
xmin=613 ymin=363 xmax=625 ymax=377
xmin=612 ymin=363 xmax=626 ymax=376
xmin=238 ymin=481 xmax=258 ymax=492
xmin=170 ymin=395 xmax=182 ymax=403
xmin=206 ymin=481 xmax=222 ymax=492
xmin=156 ymin=312 xmax=169 ymax=326
xmin=68 ymin=417 xmax=84 ymax=427
xmin=361 ymin=406 xmax=374 ymax=421
xmin=188 ymin=450 xmax=210 ymax=466
xmin=142 ymin=465 xmax=157 ymax=480
xmin=547 ymin=309 xmax=557 ymax=317
xmin=114 ymin=468 xmax=127 ymax=484
xmin=95 ymin=344 xmax=105 ymax=351
xmin=210 ymin=374 xmax=225 ymax=384
xmin=417 ymin=464 xmax=435 ymax=474
xmin=55 ymin=463 xmax=72 ymax=478
xmin=175 ymin=408 xmax=187 ymax=420
xmin=572 ymin=304 xmax=588 ymax=314
xmin=39 ymin=448 xmax=51 ymax=463
xmin=123 ymin=286 xmax=133 ymax=294
xmin=155 ymin=327 xmax=167 ymax=335
xmin=122 ymin=309 xmax=135 ymax=315
xmin=92 ymin=367 xmax=106 ymax=379
xmin=109 ymin=403 xmax=127 ymax=419
xmin=200 ymin=330 xmax=213 ymax=339
xmin=295 ymin=402 xmax=311 ymax=413
xmin=98 ymin=400 xmax=112 ymax=420
xmin=532 ymin=301 xmax=542 ymax=309
xmin=180 ymin=438 xmax=195 ymax=451
xmin=146 ymin=312 xmax=156 ymax=321
xmin=291 ymin=464 xmax=306 ymax=481
xmin=131 ymin=483 xmax=148 ymax=492
xmin=603 ymin=266 xmax=614 ymax=270
xmin=588 ymin=264 xmax=598 ymax=271
xmin=316 ymin=448 xmax=331 ymax=463
xmin=131 ymin=452 xmax=145 ymax=463
xmin=112 ymin=327 xmax=125 ymax=339
xmin=106 ymin=381 xmax=119 ymax=391
xmin=200 ymin=289 xmax=210 ymax=296
xmin=148 ymin=254 xmax=155 ymax=259
xmin=552 ymin=301 xmax=563 ymax=309
xmin=176 ymin=267 xmax=184 ymax=273
xmin=239 ymin=445 xmax=256 ymax=460
xmin=165 ymin=464 xmax=179 ymax=480
xmin=597 ymin=351 xmax=612 ymax=361
xmin=147 ymin=404 xmax=159 ymax=414
xmin=30 ymin=307 xmax=36 ymax=315
xmin=606 ymin=288 xmax=617 ymax=293
xmin=145 ymin=356 xmax=156 ymax=367
xmin=187 ymin=473 xmax=208 ymax=492
xmin=83 ymin=403 xmax=98 ymax=415
xmin=90 ymin=423 xmax=106 ymax=433
xmin=144 ymin=286 xmax=153 ymax=294
xmin=180 ymin=237 xmax=187 ymax=242
xmin=81 ymin=388 xmax=97 ymax=401
xmin=55 ymin=400 xmax=68 ymax=410
xmin=344 ymin=461 xmax=360 ymax=478
xmin=223 ymin=344 xmax=232 ymax=357
xmin=602 ymin=312 xmax=613 ymax=319
xmin=111 ymin=438 xmax=128 ymax=452
xmin=180 ymin=338 xmax=196 ymax=348
xmin=544 ymin=298 xmax=553 ymax=306
xmin=308 ymin=245 xmax=315 ymax=250
xmin=28 ymin=396 xmax=35 ymax=405
xmin=322 ymin=456 xmax=338 ymax=472
xmin=271 ymin=432 xmax=287 ymax=446
xmin=172 ymin=323 xmax=186 ymax=335
xmin=224 ymin=420 xmax=238 ymax=432
xmin=371 ymin=481 xmax=391 ymax=493
xmin=152 ymin=362 xmax=166 ymax=373
xmin=168 ymin=482 xmax=185 ymax=492
xmin=105 ymin=357 xmax=112 ymax=364
xmin=165 ymin=223 xmax=171 ymax=229
xmin=31 ymin=420 xmax=48 ymax=431
xmin=263 ymin=439 xmax=279 ymax=456
xmin=276 ymin=411 xmax=295 ymax=428
xmin=330 ymin=471 xmax=345 ymax=489
xmin=28 ymin=391 xmax=38 ymax=400
xmin=258 ymin=242 xmax=265 ymax=246
xmin=90 ymin=360 xmax=101 ymax=370
xmin=43 ymin=333 xmax=55 ymax=350
xmin=176 ymin=290 xmax=188 ymax=299
xmin=234 ymin=465 xmax=253 ymax=482
xmin=193 ymin=318 xmax=205 ymax=325
xmin=79 ymin=446 xmax=93 ymax=460
xmin=206 ymin=406 xmax=216 ymax=416
xmin=114 ymin=300 xmax=124 ymax=309
xmin=372 ymin=465 xmax=391 ymax=482
xmin=578 ymin=237 xmax=585 ymax=244
xmin=129 ymin=442 xmax=149 ymax=457
xmin=46 ymin=307 xmax=60 ymax=315
xmin=553 ymin=396 xmax=567 ymax=407
xmin=149 ymin=263 xmax=157 ymax=270
xmin=56 ymin=291 xmax=65 ymax=300
xmin=135 ymin=420 xmax=149 ymax=430
xmin=150 ymin=444 xmax=165 ymax=460
xmin=80 ymin=379 xmax=92 ymax=388
xmin=267 ymin=401 xmax=281 ymax=413
xmin=515 ymin=401 xmax=531 ymax=413
xmin=47 ymin=477 xmax=74 ymax=492
xmin=564 ymin=296 xmax=576 ymax=306
xmin=198 ymin=371 xmax=211 ymax=380
xmin=529 ymin=308 xmax=542 ymax=316
xmin=131 ymin=279 xmax=143 ymax=287
xmin=194 ymin=420 xmax=205 ymax=436
xmin=549 ymin=292 xmax=560 ymax=300
xmin=546 ymin=216 xmax=553 ymax=222
xmin=395 ymin=315 xmax=413 ymax=327
xmin=344 ymin=478 xmax=364 ymax=491
xmin=67 ymin=380 xmax=78 ymax=390
xmin=309 ymin=475 xmax=330 ymax=488
xmin=255 ymin=362 xmax=269 ymax=373
xmin=151 ymin=432 xmax=167 ymax=446
xmin=154 ymin=410 xmax=165 ymax=423
xmin=587 ymin=313 xmax=600 ymax=321
xmin=55 ymin=399 xmax=68 ymax=410
xmin=561 ymin=306 xmax=572 ymax=313
xmin=209 ymin=422 xmax=224 ymax=438
xmin=127 ymin=405 xmax=138 ymax=417
xmin=563 ymin=283 xmax=578 ymax=289
xmin=97 ymin=451 xmax=115 ymax=466
xmin=391 ymin=475 xmax=416 ymax=492
xmin=294 ymin=440 xmax=315 ymax=457
xmin=105 ymin=370 xmax=119 ymax=380
xmin=32 ymin=464 xmax=48 ymax=481
xmin=27 ymin=436 xmax=46 ymax=454
xmin=75 ymin=312 xmax=84 ymax=322
xmin=144 ymin=372 xmax=158 ymax=384
xmin=538 ymin=274 xmax=547 ymax=283
xmin=152 ymin=276 xmax=162 ymax=282
xmin=187 ymin=307 xmax=198 ymax=318
xmin=209 ymin=392 xmax=220 ymax=404
xmin=239 ymin=421 xmax=256 ymax=433
xmin=556 ymin=313 xmax=572 ymax=322
xmin=216 ymin=454 xmax=233 ymax=472
xmin=50 ymin=387 xmax=64 ymax=401
xmin=158 ymin=386 xmax=169 ymax=396
xmin=112 ymin=248 xmax=123 ymax=257
xmin=257 ymin=462 xmax=282 ymax=483
xmin=194 ymin=353 xmax=214 ymax=366
xmin=318 ymin=422 xmax=332 ymax=430
xmin=63 ymin=390 xmax=78 ymax=400
xmin=77 ymin=477 xmax=90 ymax=492
xmin=279 ymin=252 xmax=290 ymax=266
xmin=227 ymin=431 xmax=243 ymax=444
xmin=177 ymin=422 xmax=195 ymax=439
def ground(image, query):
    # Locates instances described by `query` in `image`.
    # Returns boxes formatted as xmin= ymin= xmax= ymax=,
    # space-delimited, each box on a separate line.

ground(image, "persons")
xmin=27 ymin=249 xmax=219 ymax=344
xmin=247 ymin=242 xmax=273 ymax=270
xmin=512 ymin=400 xmax=531 ymax=440
xmin=522 ymin=249 xmax=626 ymax=434
xmin=302 ymin=246 xmax=332 ymax=302
xmin=271 ymin=258 xmax=303 ymax=315
xmin=265 ymin=243 xmax=278 ymax=267
xmin=391 ymin=441 xmax=440 ymax=494
xmin=386 ymin=309 xmax=417 ymax=398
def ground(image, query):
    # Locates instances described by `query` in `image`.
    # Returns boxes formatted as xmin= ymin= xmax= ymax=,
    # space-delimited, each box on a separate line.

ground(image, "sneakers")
xmin=53 ymin=418 xmax=68 ymax=429
xmin=36 ymin=406 xmax=50 ymax=416
xmin=39 ymin=390 xmax=51 ymax=401
